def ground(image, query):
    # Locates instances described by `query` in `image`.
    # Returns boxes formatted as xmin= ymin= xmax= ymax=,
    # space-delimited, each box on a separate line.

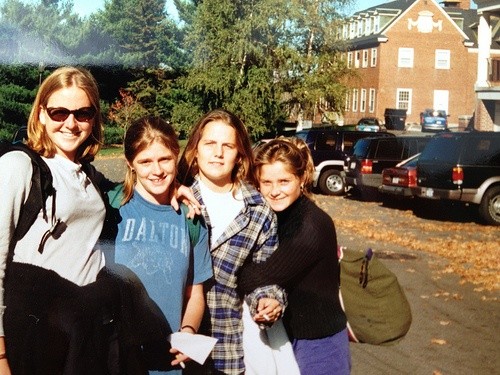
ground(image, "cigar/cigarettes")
xmin=264 ymin=314 xmax=270 ymax=321
xmin=192 ymin=204 xmax=207 ymax=208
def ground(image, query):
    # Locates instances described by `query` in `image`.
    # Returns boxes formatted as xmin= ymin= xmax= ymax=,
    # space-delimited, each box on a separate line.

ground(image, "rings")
xmin=176 ymin=355 xmax=185 ymax=368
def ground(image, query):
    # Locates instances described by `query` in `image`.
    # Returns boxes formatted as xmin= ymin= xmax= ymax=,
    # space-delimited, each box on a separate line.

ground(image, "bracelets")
xmin=178 ymin=325 xmax=197 ymax=334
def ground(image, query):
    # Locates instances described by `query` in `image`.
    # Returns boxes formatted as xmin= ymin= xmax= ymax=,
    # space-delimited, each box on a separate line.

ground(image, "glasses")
xmin=45 ymin=106 xmax=96 ymax=121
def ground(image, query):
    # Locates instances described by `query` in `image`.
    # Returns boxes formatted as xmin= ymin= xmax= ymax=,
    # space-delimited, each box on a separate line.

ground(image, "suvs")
xmin=306 ymin=125 xmax=395 ymax=196
xmin=415 ymin=130 xmax=500 ymax=226
xmin=295 ymin=130 xmax=310 ymax=143
xmin=345 ymin=134 xmax=434 ymax=202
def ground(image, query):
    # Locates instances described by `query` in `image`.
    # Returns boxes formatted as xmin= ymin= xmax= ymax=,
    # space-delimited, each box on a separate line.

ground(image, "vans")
xmin=422 ymin=108 xmax=450 ymax=133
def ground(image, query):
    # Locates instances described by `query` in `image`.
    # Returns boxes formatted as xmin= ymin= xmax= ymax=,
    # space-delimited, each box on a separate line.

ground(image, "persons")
xmin=174 ymin=111 xmax=289 ymax=375
xmin=100 ymin=116 xmax=214 ymax=375
xmin=0 ymin=65 xmax=202 ymax=375
xmin=236 ymin=138 xmax=352 ymax=375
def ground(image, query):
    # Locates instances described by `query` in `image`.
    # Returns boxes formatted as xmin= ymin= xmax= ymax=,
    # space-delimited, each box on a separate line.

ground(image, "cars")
xmin=377 ymin=153 xmax=423 ymax=208
xmin=355 ymin=117 xmax=387 ymax=133
